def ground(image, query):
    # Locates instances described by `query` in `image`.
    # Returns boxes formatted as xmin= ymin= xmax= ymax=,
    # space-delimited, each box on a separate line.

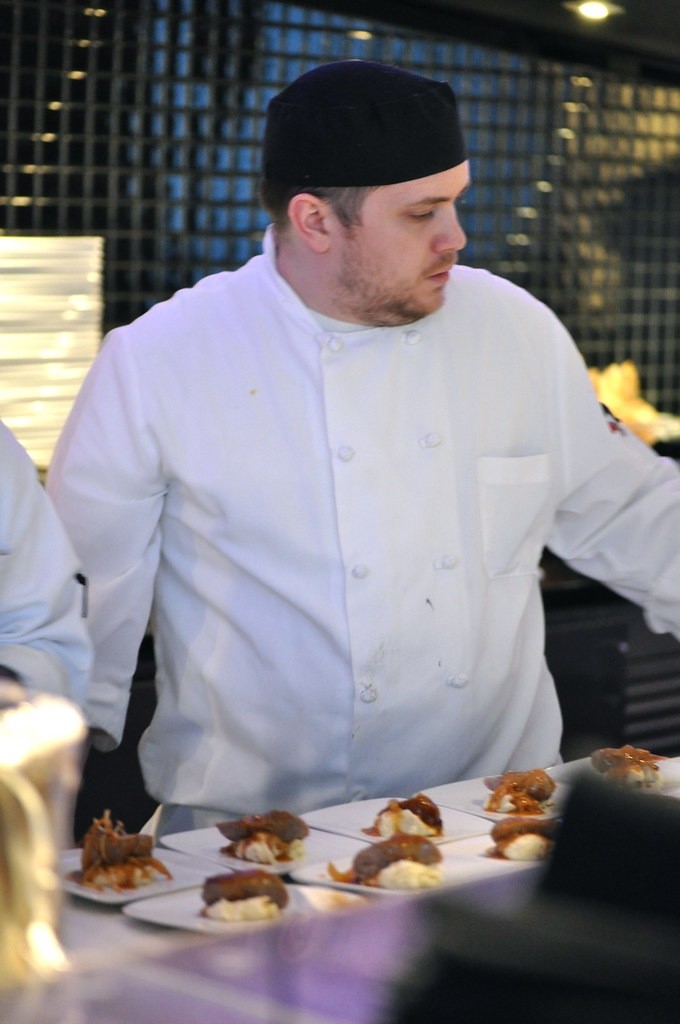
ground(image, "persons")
xmin=46 ymin=61 xmax=680 ymax=841
xmin=0 ymin=423 xmax=96 ymax=711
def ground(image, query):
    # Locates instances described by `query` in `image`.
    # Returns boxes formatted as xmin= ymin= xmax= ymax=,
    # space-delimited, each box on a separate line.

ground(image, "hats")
xmin=263 ymin=59 xmax=469 ymax=187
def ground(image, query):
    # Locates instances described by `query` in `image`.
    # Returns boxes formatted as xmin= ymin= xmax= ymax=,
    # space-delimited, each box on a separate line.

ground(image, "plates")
xmin=299 ymin=796 xmax=497 ymax=845
xmin=287 ymin=848 xmax=539 ymax=896
xmin=121 ymin=883 xmax=365 ymax=931
xmin=413 ymin=772 xmax=570 ymax=821
xmin=52 ymin=847 xmax=234 ymax=905
xmin=158 ymin=827 xmax=373 ymax=873
xmin=545 ymin=756 xmax=671 ymax=789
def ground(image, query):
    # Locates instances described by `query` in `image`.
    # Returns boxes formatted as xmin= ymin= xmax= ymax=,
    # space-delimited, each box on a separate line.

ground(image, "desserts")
xmin=202 ymin=867 xmax=289 ymax=923
xmin=79 ymin=809 xmax=174 ymax=891
xmin=217 ymin=808 xmax=309 ymax=864
xmin=324 ymin=746 xmax=663 ymax=890
